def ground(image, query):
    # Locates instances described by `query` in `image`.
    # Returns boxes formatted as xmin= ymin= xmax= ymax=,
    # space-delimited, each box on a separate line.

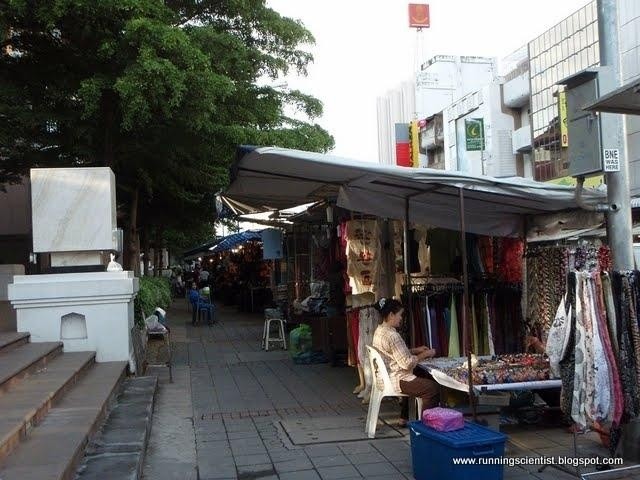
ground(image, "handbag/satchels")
xmin=422 ymin=407 xmax=464 ymax=433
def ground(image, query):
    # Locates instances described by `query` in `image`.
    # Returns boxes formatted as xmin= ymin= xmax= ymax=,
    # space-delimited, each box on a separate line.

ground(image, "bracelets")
xmin=411 ymin=348 xmax=414 ymax=354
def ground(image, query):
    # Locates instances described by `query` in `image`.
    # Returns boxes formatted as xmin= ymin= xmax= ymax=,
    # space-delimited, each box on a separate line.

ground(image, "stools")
xmin=262 ymin=317 xmax=287 ymax=351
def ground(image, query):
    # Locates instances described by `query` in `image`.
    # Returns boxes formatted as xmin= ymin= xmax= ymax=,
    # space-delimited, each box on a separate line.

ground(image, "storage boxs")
xmin=408 ymin=416 xmax=509 ymax=479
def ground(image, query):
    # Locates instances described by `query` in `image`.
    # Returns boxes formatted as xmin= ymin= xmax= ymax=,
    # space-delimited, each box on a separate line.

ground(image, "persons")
xmin=171 ymin=264 xmax=217 ymax=325
xmin=342 ymin=269 xmax=382 ymax=404
xmin=372 ymin=298 xmax=439 ymax=427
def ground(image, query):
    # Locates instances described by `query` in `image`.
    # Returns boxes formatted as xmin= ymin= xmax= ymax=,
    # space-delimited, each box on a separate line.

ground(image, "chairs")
xmin=362 ymin=344 xmax=423 ymax=439
